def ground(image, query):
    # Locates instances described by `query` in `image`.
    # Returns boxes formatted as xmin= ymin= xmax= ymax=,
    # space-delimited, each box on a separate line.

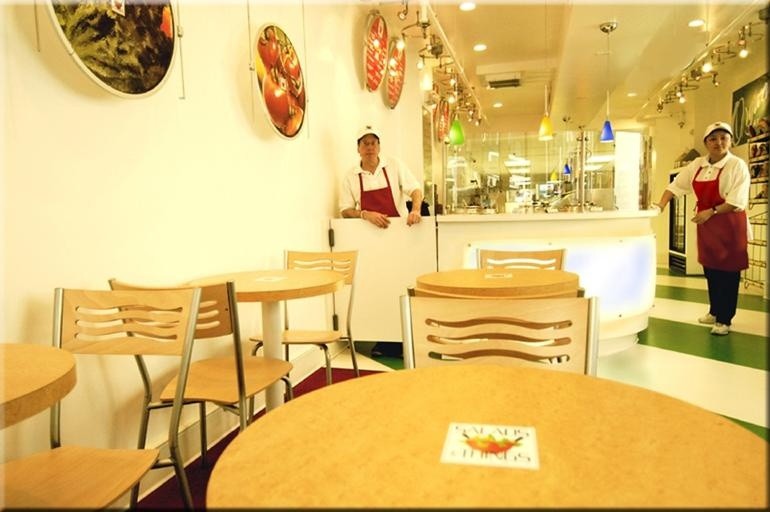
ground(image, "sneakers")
xmin=700 ymin=313 xmax=716 ymax=324
xmin=711 ymin=323 xmax=729 ymax=335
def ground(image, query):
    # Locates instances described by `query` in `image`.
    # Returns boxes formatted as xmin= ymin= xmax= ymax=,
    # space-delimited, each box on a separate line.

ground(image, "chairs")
xmin=393 ymin=292 xmax=604 ymax=378
xmin=475 ymin=245 xmax=568 ymax=274
xmin=105 ymin=274 xmax=299 ymax=465
xmin=1 ymin=282 xmax=206 ymax=512
xmin=248 ymin=246 xmax=362 ymax=398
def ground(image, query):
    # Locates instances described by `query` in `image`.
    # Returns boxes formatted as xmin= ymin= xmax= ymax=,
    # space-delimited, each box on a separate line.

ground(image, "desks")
xmin=203 ymin=363 xmax=769 ymax=512
xmin=411 ymin=269 xmax=583 ymax=302
xmin=2 ymin=342 xmax=81 ymax=431
xmin=186 ymin=264 xmax=348 ymax=416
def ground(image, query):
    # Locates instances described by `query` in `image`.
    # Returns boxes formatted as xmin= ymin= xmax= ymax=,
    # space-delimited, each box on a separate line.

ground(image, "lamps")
xmin=656 ymin=18 xmax=767 ymax=116
xmin=538 ymin=4 xmax=555 ymax=143
xmin=446 ymin=3 xmax=467 ymax=149
xmin=393 ymin=1 xmax=491 ymax=127
xmin=597 ymin=21 xmax=618 ymax=145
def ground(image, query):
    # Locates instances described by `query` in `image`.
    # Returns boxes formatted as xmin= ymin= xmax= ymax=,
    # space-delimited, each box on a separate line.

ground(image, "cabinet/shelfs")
xmin=736 ymin=131 xmax=770 ymax=303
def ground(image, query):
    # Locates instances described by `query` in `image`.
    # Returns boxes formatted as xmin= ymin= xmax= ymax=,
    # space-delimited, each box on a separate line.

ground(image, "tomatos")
xmin=257 ymin=26 xmax=304 ymax=136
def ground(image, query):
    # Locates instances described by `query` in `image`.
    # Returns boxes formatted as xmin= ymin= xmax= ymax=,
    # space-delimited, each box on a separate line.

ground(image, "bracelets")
xmin=360 ymin=209 xmax=367 ymax=219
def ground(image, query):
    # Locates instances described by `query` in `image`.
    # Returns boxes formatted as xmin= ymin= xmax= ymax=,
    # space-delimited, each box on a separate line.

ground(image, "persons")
xmin=338 ymin=122 xmax=424 ymax=229
xmin=650 ymin=120 xmax=755 ymax=336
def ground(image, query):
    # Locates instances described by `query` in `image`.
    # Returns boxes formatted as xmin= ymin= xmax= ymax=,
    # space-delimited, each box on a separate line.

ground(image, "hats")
xmin=704 ymin=122 xmax=733 ymax=139
xmin=359 ymin=127 xmax=380 ymax=140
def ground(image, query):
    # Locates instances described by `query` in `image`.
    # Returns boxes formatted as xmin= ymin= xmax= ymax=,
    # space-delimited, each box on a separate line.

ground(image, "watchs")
xmin=711 ymin=206 xmax=720 ymax=215
